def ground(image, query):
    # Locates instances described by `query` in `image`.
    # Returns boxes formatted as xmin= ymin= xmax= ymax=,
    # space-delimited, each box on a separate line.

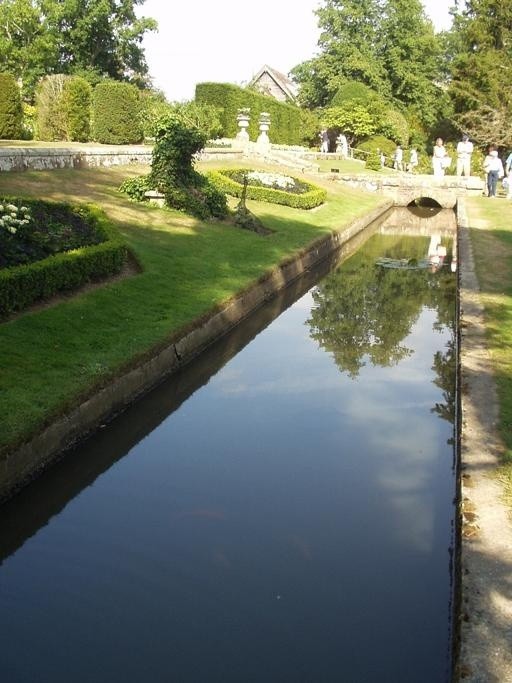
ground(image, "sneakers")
xmin=488 ymin=191 xmax=496 ymax=197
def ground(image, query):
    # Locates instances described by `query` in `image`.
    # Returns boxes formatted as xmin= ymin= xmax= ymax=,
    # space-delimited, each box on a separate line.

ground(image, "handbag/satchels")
xmin=440 ymin=155 xmax=452 ymax=168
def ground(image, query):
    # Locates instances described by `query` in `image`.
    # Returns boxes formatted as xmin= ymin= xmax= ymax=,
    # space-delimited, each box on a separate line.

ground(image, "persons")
xmin=450 ymin=233 xmax=458 ymax=273
xmin=427 ymin=235 xmax=445 ymax=275
xmin=318 ymin=127 xmax=512 ymax=199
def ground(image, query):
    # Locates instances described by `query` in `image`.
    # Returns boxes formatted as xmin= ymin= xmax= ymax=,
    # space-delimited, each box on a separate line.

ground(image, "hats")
xmin=462 ymin=135 xmax=469 ymax=140
xmin=489 ymin=150 xmax=499 ymax=157
